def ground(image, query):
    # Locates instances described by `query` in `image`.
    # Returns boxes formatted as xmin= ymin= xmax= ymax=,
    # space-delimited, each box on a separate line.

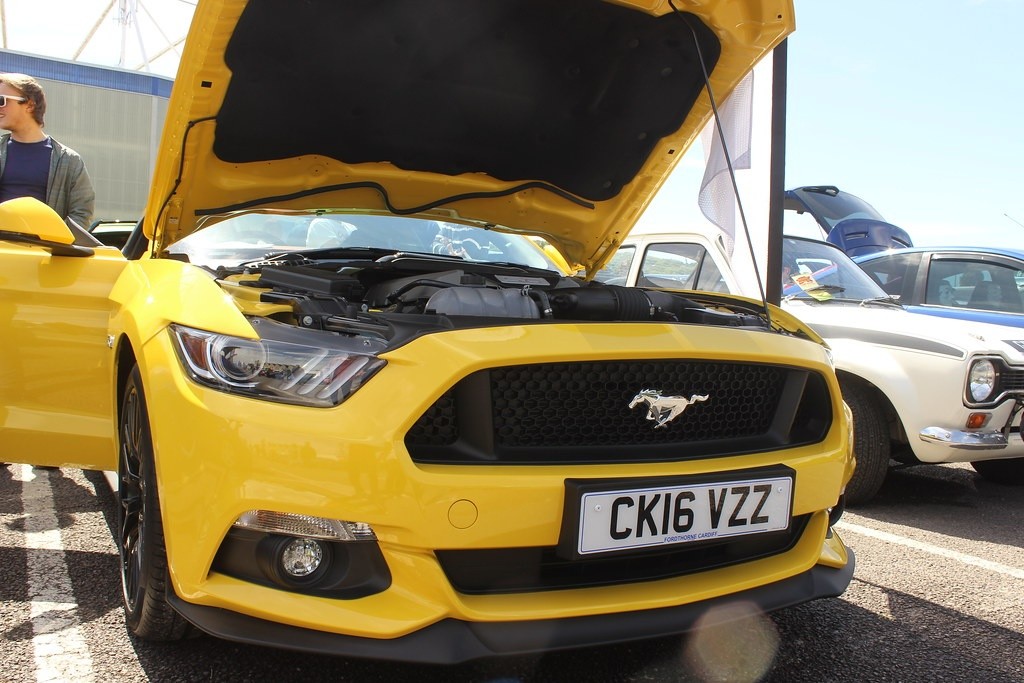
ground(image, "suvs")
xmin=591 ymin=229 xmax=1024 ymax=508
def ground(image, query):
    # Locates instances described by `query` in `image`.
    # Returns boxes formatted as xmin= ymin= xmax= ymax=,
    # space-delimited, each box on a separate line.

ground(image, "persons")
xmin=782 ymin=254 xmax=801 ymax=289
xmin=931 ymin=280 xmax=956 ymax=307
xmin=0 ymin=73 xmax=95 ymax=471
xmin=967 ymin=281 xmax=1004 ymax=308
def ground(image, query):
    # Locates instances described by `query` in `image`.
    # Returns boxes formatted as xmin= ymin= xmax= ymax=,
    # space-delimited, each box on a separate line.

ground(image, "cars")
xmin=0 ymin=1 xmax=853 ymax=669
xmin=784 ymin=185 xmax=1024 ymax=328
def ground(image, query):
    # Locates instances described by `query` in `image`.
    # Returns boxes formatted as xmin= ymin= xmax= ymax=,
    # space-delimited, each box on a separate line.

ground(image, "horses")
xmin=629 ymin=388 xmax=709 ymax=429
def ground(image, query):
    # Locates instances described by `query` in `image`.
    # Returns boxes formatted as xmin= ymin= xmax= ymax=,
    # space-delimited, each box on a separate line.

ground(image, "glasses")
xmin=0 ymin=93 xmax=28 ymax=107
